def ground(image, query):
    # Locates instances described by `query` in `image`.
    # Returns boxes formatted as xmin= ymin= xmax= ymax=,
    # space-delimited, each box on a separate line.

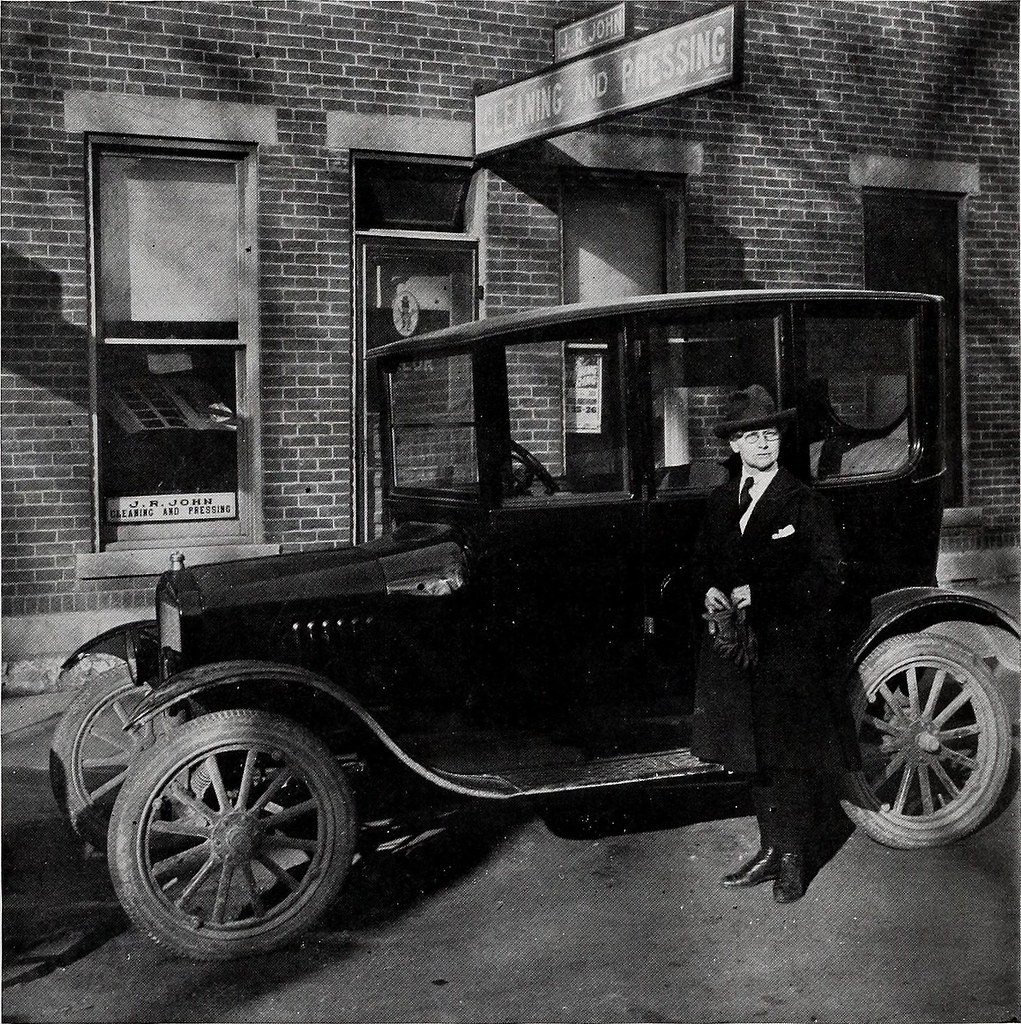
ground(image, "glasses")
xmin=744 ymin=432 xmax=781 ymax=444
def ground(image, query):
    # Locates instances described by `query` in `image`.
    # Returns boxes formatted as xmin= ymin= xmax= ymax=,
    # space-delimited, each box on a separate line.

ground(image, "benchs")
xmin=806 ymin=434 xmax=910 ymax=478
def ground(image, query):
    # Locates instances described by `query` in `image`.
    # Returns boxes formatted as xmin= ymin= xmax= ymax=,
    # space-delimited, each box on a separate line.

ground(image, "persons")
xmin=689 ymin=385 xmax=863 ymax=903
xmin=397 ymin=297 xmax=417 ymax=332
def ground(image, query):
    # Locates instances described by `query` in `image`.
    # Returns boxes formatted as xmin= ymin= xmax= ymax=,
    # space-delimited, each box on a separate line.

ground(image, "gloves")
xmin=708 ymin=608 xmax=759 ymax=679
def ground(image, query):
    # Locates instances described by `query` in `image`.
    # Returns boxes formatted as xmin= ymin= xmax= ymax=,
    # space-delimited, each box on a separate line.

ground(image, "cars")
xmin=49 ymin=280 xmax=1020 ymax=957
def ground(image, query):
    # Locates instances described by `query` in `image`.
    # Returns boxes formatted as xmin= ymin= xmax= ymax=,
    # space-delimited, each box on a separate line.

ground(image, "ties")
xmin=736 ymin=477 xmax=754 ymax=523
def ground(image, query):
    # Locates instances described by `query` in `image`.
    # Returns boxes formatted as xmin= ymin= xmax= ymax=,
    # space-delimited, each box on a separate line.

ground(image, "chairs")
xmin=658 ymin=463 xmax=731 ymax=491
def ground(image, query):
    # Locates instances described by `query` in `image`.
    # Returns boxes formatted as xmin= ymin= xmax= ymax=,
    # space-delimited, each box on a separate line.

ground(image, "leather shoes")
xmin=719 ymin=847 xmax=806 ymax=903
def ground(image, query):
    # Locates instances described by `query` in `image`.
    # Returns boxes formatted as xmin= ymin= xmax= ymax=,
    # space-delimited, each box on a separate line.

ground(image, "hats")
xmin=713 ymin=383 xmax=798 ymax=441
